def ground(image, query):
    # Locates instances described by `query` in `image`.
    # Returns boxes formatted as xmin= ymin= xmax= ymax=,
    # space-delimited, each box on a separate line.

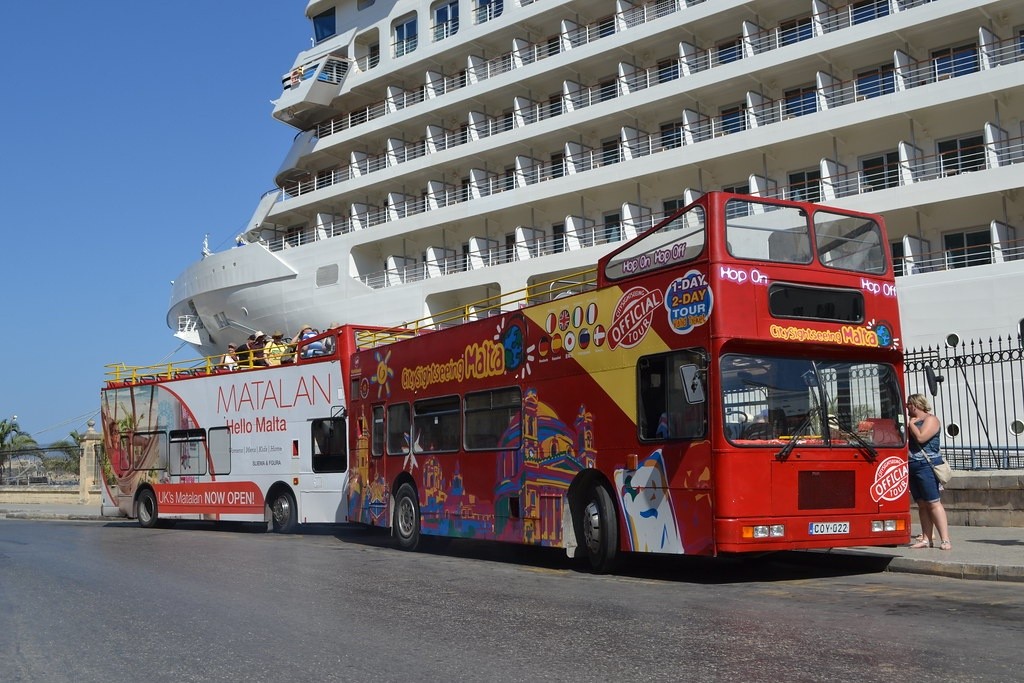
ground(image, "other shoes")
xmin=910 ymin=540 xmax=933 ymax=548
xmin=940 ymin=539 xmax=951 ymax=550
xmin=916 ymin=534 xmax=936 ymax=541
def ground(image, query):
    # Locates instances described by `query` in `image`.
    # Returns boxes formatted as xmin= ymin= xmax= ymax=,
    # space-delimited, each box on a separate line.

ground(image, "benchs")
xmin=396 ymin=292 xmax=576 ymax=343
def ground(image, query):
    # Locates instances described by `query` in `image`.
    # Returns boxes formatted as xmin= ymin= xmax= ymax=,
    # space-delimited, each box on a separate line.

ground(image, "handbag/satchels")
xmin=933 ymin=458 xmax=952 ymax=484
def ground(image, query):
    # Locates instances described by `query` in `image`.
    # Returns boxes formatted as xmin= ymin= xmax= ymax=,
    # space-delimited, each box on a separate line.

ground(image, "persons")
xmin=906 ymin=393 xmax=952 ymax=551
xmin=220 ymin=321 xmax=340 ymax=369
xmin=655 ymin=413 xmax=668 ymax=438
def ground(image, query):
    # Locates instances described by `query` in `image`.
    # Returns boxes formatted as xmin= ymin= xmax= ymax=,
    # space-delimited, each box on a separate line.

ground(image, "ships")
xmin=164 ymin=1 xmax=1024 ymax=469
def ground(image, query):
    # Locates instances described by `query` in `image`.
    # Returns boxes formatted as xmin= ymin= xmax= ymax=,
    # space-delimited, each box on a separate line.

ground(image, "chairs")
xmin=106 ymin=364 xmax=257 ymax=389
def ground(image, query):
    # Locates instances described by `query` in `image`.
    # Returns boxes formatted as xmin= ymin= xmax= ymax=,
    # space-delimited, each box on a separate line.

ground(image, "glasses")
xmin=906 ymin=403 xmax=910 ymax=407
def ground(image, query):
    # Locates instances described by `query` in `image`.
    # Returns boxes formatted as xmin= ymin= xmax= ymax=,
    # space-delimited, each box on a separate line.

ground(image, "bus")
xmin=100 ymin=324 xmax=439 ymax=535
xmin=347 ymin=187 xmax=946 ymax=575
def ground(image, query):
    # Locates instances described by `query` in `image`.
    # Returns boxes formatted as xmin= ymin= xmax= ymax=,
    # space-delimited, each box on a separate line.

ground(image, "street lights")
xmin=9 ymin=415 xmax=18 ymax=485
